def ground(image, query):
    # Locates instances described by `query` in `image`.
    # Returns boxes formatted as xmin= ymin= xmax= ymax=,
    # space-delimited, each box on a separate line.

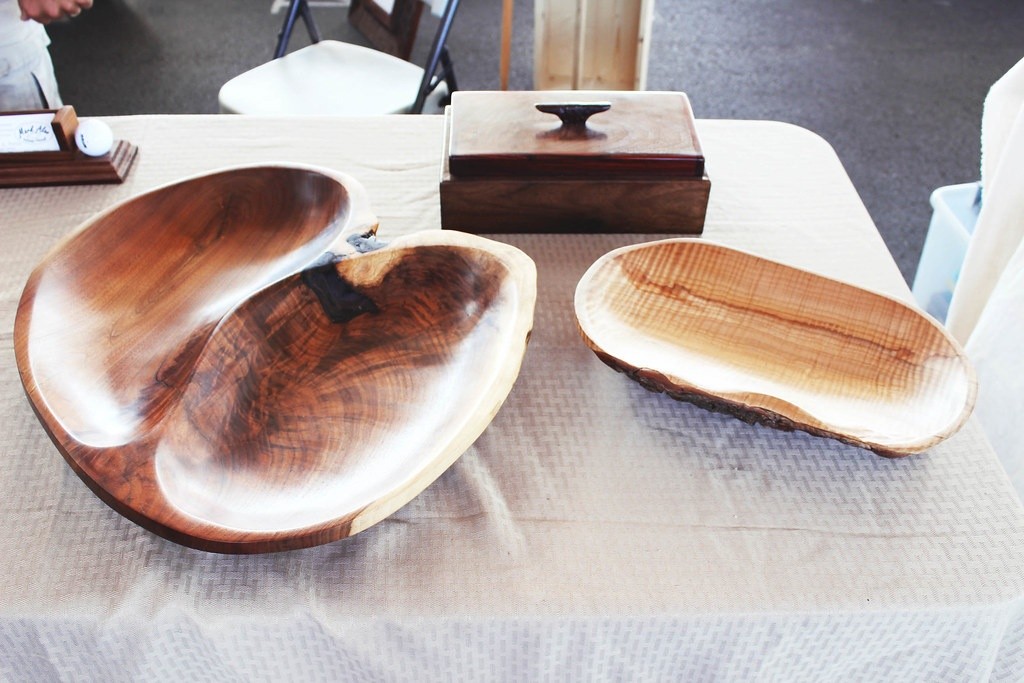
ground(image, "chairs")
xmin=216 ymin=0 xmax=463 ymax=114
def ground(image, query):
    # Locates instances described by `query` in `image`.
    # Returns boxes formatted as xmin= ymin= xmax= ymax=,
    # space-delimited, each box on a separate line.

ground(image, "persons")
xmin=0 ymin=0 xmax=93 ymax=112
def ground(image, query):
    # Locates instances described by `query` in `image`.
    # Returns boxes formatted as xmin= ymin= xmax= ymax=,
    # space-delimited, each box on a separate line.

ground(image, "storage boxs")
xmin=441 ymin=90 xmax=710 ymax=235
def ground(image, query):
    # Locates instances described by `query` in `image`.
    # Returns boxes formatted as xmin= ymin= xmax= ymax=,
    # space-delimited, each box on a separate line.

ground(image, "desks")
xmin=0 ymin=111 xmax=1024 ymax=683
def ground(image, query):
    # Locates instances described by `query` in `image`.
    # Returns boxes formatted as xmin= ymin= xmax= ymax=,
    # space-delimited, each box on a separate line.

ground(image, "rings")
xmin=70 ymin=8 xmax=81 ymax=18
